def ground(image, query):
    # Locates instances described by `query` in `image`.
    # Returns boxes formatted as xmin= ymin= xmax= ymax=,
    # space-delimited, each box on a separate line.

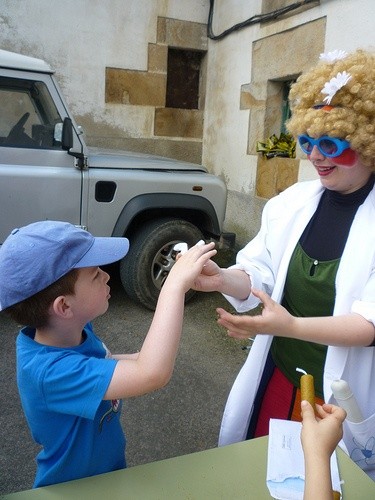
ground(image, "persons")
xmin=296 ymin=401 xmax=348 ymax=499
xmin=0 ymin=222 xmax=218 ymax=488
xmin=174 ymin=48 xmax=374 ymax=488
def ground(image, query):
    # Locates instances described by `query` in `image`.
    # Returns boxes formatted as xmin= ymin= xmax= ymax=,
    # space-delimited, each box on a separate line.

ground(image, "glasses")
xmin=298 ymin=133 xmax=351 ymax=158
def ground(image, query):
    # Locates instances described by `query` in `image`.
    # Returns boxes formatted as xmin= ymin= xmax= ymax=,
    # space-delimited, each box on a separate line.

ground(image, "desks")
xmin=0 ymin=434 xmax=375 ymax=500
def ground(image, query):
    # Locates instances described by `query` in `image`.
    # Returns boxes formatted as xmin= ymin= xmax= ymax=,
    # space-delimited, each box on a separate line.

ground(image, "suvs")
xmin=1 ymin=50 xmax=237 ymax=311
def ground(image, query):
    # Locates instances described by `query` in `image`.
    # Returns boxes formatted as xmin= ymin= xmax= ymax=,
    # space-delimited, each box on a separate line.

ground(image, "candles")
xmin=296 ymin=369 xmax=319 ymax=413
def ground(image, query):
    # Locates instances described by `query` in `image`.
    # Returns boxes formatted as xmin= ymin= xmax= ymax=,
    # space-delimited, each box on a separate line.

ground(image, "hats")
xmin=0 ymin=221 xmax=130 ymax=309
